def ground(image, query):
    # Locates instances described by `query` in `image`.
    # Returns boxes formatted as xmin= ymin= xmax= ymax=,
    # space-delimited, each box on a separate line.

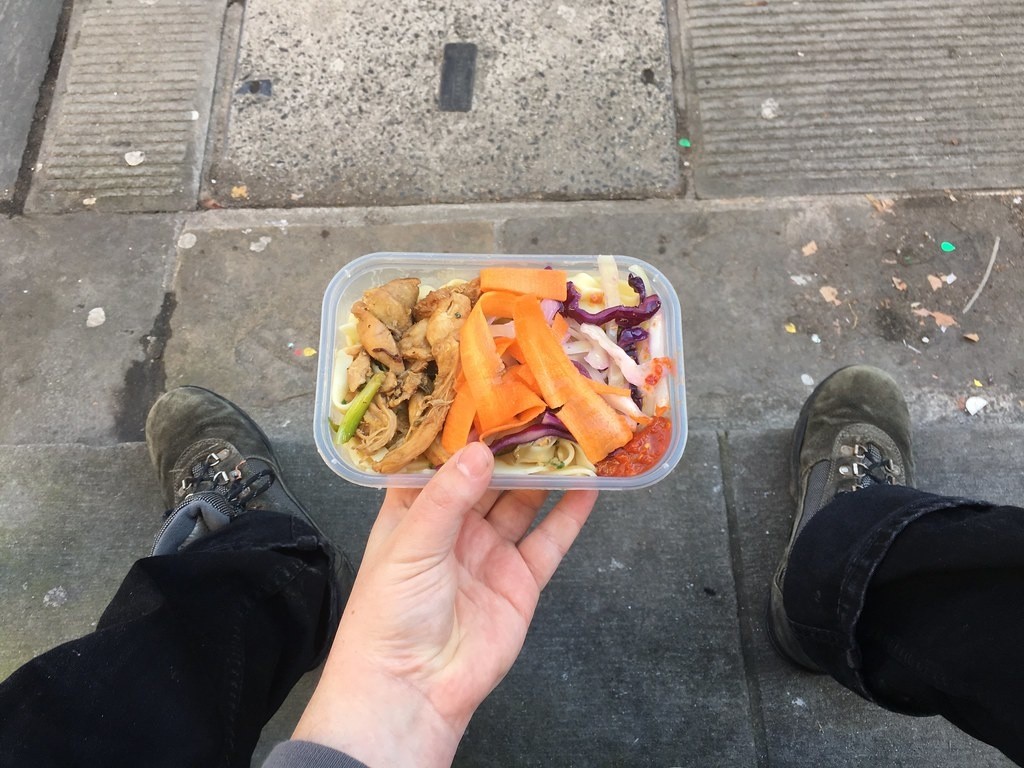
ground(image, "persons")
xmin=0 ymin=364 xmax=1024 ymax=768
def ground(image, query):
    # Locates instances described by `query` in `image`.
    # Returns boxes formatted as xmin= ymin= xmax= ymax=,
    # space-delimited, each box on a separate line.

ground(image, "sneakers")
xmin=140 ymin=386 xmax=355 ymax=673
xmin=768 ymin=361 xmax=919 ymax=674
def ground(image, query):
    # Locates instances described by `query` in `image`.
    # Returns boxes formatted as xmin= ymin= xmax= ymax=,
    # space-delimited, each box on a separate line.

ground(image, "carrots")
xmin=442 ymin=267 xmax=633 ymax=464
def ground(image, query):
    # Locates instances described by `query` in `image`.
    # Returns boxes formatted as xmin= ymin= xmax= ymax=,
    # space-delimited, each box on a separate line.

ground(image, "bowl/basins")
xmin=313 ymin=250 xmax=689 ymax=493
